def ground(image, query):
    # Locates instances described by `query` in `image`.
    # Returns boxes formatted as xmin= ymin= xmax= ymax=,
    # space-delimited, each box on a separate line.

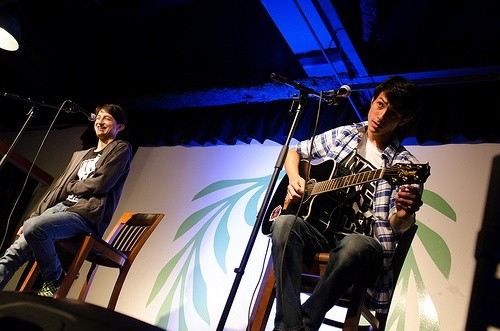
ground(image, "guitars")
xmin=261 ymin=158 xmax=430 ymax=238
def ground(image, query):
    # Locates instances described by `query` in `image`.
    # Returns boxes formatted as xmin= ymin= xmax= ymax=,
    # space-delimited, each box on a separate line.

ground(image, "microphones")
xmin=321 ymin=84 xmax=351 ymax=98
xmin=70 ymin=100 xmax=96 ymax=121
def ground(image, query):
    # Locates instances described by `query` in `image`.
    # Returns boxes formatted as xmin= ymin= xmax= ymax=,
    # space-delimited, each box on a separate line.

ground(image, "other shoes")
xmin=37 ymin=279 xmax=60 ymax=298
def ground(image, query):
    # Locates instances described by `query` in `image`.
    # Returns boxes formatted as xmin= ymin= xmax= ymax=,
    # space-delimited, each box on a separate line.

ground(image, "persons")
xmin=0 ymin=104 xmax=135 ymax=297
xmin=270 ymin=76 xmax=424 ymax=331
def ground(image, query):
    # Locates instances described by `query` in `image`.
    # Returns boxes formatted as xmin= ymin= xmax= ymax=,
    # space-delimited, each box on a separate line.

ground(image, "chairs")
xmin=19 ymin=213 xmax=165 ymax=310
xmin=245 ymin=221 xmax=418 ymax=331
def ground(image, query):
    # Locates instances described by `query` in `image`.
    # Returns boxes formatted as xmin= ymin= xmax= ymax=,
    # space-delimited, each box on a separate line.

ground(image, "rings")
xmin=407 ymin=205 xmax=412 ymax=211
